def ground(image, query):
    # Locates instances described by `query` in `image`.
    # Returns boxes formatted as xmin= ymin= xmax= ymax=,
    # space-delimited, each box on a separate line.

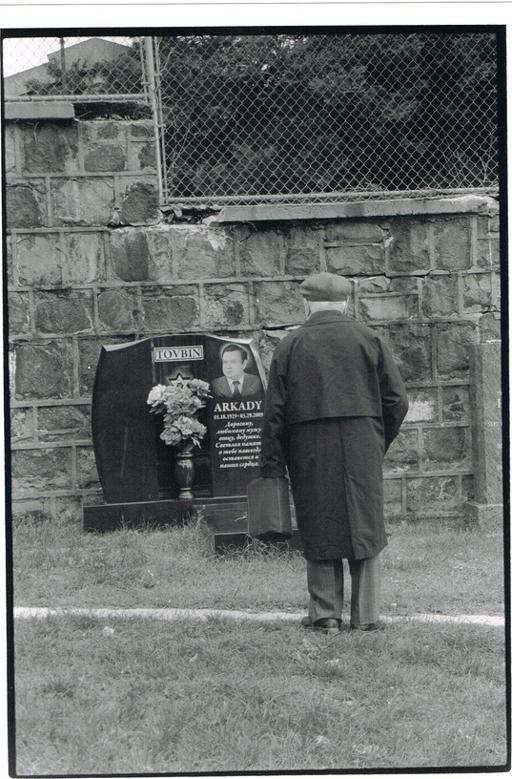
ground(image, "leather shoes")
xmin=301 ymin=615 xmax=342 ymax=630
xmin=350 ymin=619 xmax=385 ymax=632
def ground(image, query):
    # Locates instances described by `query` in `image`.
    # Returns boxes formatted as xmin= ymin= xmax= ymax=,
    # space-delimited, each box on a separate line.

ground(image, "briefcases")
xmin=247 ymin=477 xmax=293 ymax=542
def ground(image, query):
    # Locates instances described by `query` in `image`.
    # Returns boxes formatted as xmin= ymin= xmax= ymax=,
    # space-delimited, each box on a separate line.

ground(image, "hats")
xmin=299 ymin=273 xmax=352 ymax=301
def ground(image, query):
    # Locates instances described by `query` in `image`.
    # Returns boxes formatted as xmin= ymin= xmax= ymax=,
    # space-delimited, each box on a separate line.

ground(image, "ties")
xmin=233 ymin=381 xmax=241 ymax=400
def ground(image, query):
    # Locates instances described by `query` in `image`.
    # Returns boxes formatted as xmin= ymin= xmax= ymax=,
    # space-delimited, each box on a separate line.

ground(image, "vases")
xmin=174 ymin=452 xmax=195 ymax=499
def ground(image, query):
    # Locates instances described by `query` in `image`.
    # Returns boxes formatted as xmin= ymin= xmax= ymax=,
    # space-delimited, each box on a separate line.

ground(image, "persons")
xmin=260 ymin=272 xmax=408 ymax=634
xmin=212 ymin=344 xmax=261 ymax=399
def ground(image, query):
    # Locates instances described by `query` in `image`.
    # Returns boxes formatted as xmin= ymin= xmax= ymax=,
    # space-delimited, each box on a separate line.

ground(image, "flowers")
xmin=147 ymin=378 xmax=214 ymax=452
